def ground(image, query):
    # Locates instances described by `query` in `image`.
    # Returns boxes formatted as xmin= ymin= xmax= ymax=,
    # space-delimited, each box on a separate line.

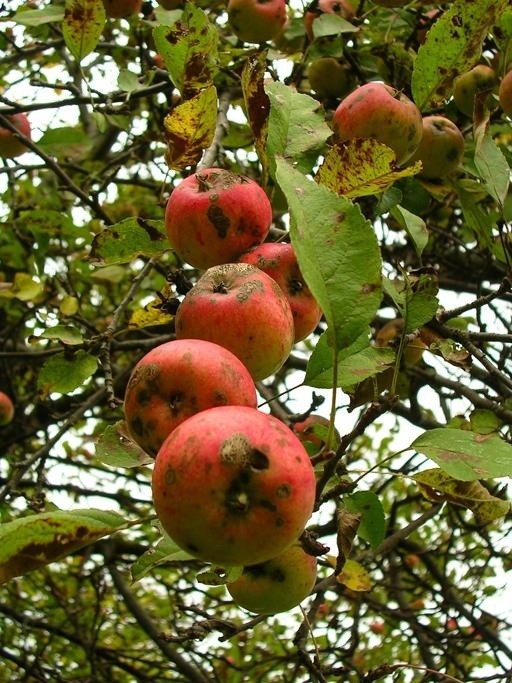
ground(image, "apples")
xmin=175 ymin=264 xmax=294 ymax=382
xmin=452 ymin=64 xmax=498 ymax=118
xmin=234 ymin=242 xmax=323 ymax=344
xmin=219 ymin=97 xmax=253 ymax=148
xmin=104 ymin=1 xmax=141 ymax=19
xmin=376 ymin=319 xmax=426 ymax=365
xmin=161 ymin=169 xmax=273 ymax=272
xmin=226 ymin=539 xmax=318 ymax=614
xmin=308 ymin=58 xmax=348 ymax=100
xmin=416 ymin=10 xmax=460 ymax=43
xmin=227 ymin=1 xmax=287 ymax=43
xmin=498 ymin=72 xmax=511 ymax=119
xmin=333 ymin=83 xmax=423 ymax=166
xmin=152 ymin=405 xmax=317 ymax=567
xmin=304 ymin=2 xmax=356 ymax=41
xmin=0 ymin=390 xmax=16 ymax=426
xmin=290 ymin=415 xmax=341 ymax=458
xmin=398 ymin=116 xmax=465 ymax=177
xmin=125 ymin=339 xmax=257 ymax=461
xmin=0 ymin=113 xmax=31 ymax=156
